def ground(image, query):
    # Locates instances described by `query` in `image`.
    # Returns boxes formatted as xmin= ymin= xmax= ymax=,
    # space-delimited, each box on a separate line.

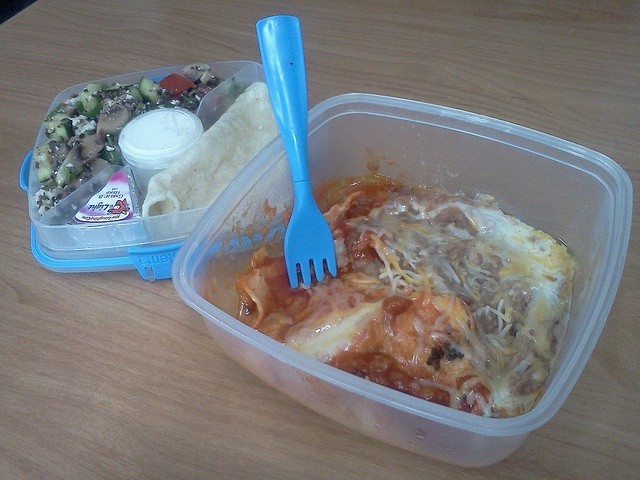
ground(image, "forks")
xmin=255 ymin=13 xmax=339 ymax=289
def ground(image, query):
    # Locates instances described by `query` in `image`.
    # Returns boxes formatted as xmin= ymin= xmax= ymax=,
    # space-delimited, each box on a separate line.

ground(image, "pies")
xmin=141 ymin=81 xmax=277 ymax=240
